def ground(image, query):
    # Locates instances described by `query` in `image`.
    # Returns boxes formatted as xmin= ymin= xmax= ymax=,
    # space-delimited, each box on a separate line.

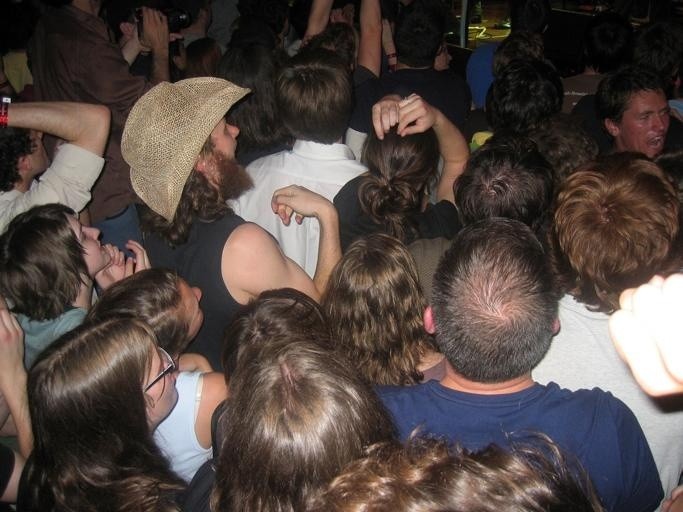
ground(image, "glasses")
xmin=142 ymin=346 xmax=175 ymax=391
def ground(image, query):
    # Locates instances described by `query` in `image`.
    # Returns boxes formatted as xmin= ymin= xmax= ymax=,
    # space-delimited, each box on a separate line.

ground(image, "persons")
xmin=0 ymin=0 xmax=682 ymax=512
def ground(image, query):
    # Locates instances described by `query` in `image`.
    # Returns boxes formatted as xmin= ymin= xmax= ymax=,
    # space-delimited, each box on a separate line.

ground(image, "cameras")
xmin=134 ymin=6 xmax=192 ymax=41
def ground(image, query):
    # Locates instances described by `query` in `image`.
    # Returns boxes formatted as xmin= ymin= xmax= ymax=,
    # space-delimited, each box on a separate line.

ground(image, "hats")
xmin=122 ymin=78 xmax=253 ymax=223
